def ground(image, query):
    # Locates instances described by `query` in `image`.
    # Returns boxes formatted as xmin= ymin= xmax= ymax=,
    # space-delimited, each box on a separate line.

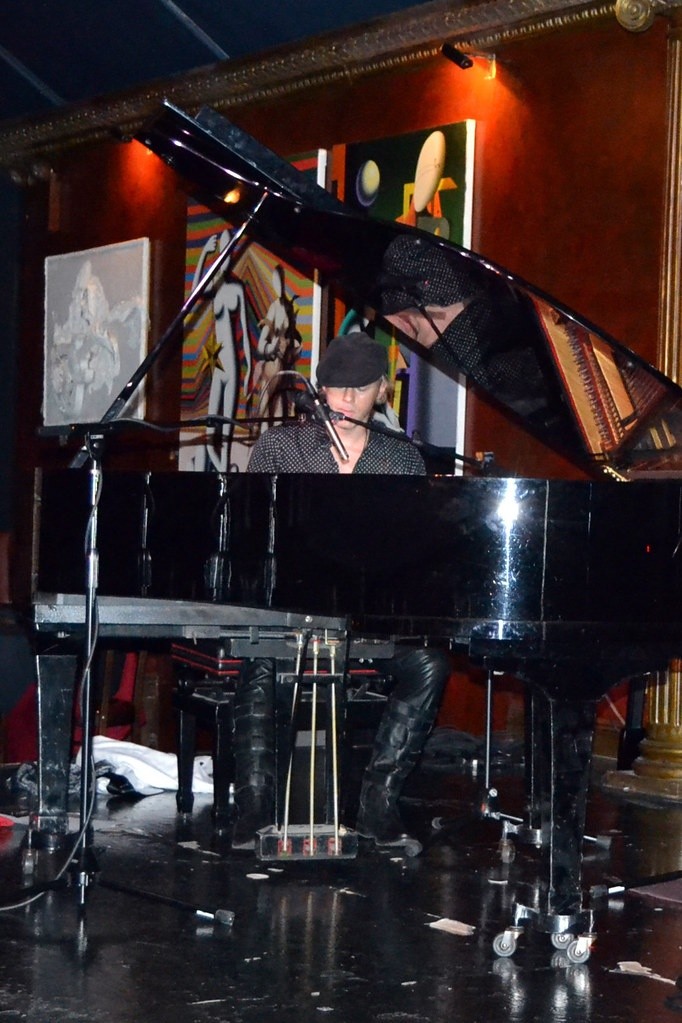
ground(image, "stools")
xmin=169 ymin=640 xmax=385 ymax=838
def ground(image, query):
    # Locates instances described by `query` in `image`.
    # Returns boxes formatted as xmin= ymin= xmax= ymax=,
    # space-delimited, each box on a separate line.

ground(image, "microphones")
xmin=477 ymin=450 xmax=499 ymax=478
xmin=302 ymin=375 xmax=349 ymax=461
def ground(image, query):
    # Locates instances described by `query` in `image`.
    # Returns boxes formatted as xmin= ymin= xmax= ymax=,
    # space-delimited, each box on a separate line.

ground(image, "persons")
xmin=380 ymin=234 xmax=534 ymax=392
xmin=234 ymin=332 xmax=449 ymax=848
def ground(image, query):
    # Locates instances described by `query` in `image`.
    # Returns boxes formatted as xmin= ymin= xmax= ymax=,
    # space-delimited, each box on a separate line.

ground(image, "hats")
xmin=316 ymin=331 xmax=389 ymax=388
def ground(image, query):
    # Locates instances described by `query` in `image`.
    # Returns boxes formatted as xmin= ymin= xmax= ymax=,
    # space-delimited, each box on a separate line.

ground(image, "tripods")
xmin=0 ymin=180 xmax=276 ymax=959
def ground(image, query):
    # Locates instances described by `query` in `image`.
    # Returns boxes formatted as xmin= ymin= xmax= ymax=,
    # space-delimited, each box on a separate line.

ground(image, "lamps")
xmin=616 ymin=0 xmax=655 ymax=33
xmin=441 ymin=42 xmax=496 ymax=79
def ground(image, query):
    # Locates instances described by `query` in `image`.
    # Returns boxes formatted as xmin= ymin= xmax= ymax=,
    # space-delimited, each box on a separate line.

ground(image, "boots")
xmin=230 ymin=659 xmax=279 ymax=848
xmin=354 ymin=696 xmax=425 ymax=856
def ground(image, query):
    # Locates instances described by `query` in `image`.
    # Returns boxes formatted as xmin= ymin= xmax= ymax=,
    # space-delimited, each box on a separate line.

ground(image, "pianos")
xmin=27 ymin=95 xmax=682 ymax=963
